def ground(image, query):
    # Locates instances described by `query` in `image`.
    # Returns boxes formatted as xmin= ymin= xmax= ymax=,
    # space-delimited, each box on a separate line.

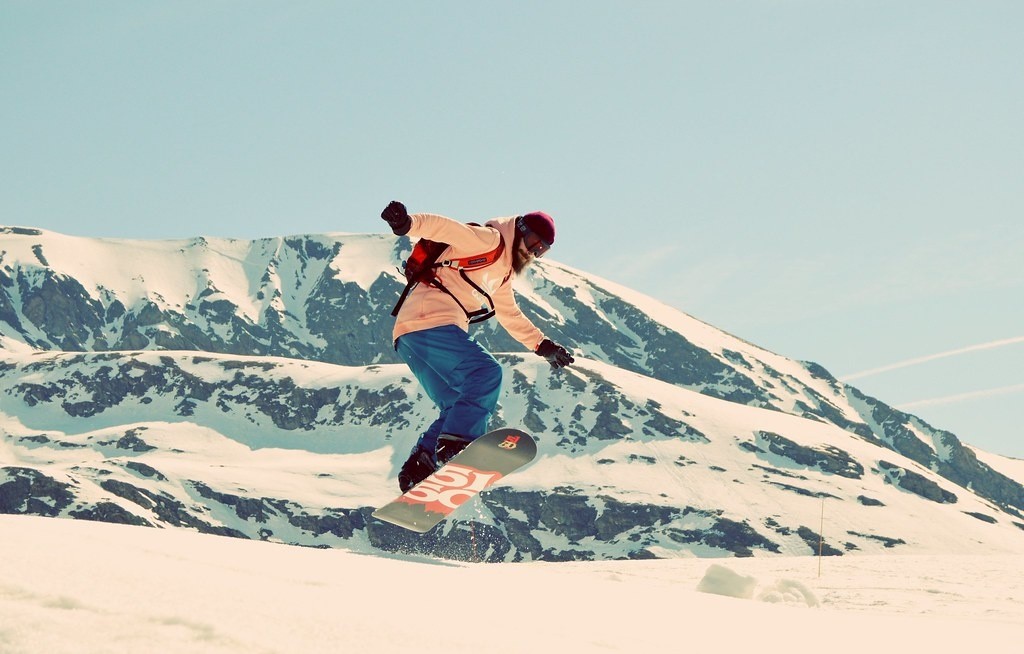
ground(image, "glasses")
xmin=523 ymin=231 xmax=550 ymax=258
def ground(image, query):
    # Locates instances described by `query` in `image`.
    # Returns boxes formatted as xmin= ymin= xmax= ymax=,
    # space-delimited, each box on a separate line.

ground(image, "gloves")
xmin=381 ymin=201 xmax=412 ymax=236
xmin=535 ymin=339 xmax=574 ymax=369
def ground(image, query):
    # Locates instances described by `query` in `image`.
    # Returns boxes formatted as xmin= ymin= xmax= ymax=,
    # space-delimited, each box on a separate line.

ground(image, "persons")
xmin=382 ymin=201 xmax=575 ymax=495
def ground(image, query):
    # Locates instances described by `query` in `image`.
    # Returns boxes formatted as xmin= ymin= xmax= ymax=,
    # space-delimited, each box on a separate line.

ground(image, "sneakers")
xmin=436 ymin=438 xmax=469 ymax=463
xmin=398 ymin=445 xmax=438 ymax=494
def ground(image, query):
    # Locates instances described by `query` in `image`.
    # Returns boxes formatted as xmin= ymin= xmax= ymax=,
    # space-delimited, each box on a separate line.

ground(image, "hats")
xmin=515 ymin=211 xmax=556 ymax=245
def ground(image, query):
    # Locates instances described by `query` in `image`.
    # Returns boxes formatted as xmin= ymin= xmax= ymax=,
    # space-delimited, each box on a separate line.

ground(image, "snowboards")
xmin=370 ymin=428 xmax=537 ymax=533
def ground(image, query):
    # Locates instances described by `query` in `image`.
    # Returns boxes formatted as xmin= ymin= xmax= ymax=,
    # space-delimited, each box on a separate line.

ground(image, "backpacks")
xmin=390 ymin=223 xmax=496 ymax=324
